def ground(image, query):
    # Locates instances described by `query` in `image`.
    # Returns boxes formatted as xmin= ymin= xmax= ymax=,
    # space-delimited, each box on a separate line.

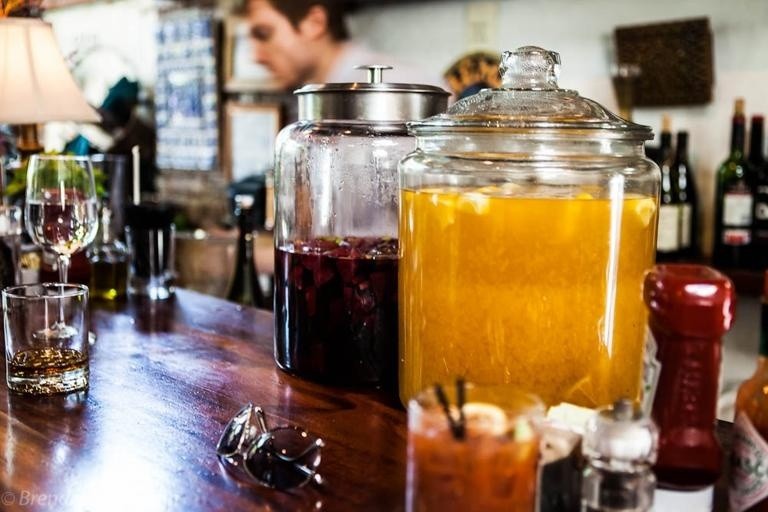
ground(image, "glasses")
xmin=217 ymin=401 xmax=330 ymax=496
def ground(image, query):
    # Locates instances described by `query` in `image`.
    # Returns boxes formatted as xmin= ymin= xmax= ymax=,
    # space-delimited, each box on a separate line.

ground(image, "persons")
xmin=221 ymin=0 xmax=458 ymax=279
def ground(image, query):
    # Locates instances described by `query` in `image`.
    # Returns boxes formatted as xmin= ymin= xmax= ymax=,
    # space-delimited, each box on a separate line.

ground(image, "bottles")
xmin=642 ymin=262 xmax=733 ymax=512
xmin=645 ymin=96 xmax=768 ymax=264
xmin=582 ymin=394 xmax=660 ymax=510
xmin=224 ymin=192 xmax=268 ymax=311
xmin=88 ymin=204 xmax=128 ymax=299
xmin=731 ymin=304 xmax=768 ymax=510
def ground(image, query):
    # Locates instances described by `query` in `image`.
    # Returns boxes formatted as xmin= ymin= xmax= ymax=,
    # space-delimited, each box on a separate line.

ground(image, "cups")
xmin=126 ymin=220 xmax=174 ymax=297
xmin=0 ymin=278 xmax=90 ymax=394
xmin=402 ymin=373 xmax=552 ymax=512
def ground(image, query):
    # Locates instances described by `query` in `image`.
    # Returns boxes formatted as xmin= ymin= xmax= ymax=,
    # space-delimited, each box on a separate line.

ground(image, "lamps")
xmin=0 ymin=15 xmax=105 ymax=251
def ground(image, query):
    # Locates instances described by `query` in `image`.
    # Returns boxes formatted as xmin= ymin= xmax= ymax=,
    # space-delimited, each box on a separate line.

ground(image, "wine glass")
xmin=28 ymin=154 xmax=100 ymax=343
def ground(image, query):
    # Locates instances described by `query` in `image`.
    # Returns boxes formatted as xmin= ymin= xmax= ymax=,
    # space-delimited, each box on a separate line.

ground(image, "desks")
xmin=1 ymin=284 xmax=412 ymax=512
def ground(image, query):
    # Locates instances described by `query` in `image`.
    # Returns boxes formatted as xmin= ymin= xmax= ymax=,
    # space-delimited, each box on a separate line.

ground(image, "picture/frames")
xmin=216 ymin=13 xmax=292 ymax=94
xmin=219 ymin=100 xmax=287 ymax=191
